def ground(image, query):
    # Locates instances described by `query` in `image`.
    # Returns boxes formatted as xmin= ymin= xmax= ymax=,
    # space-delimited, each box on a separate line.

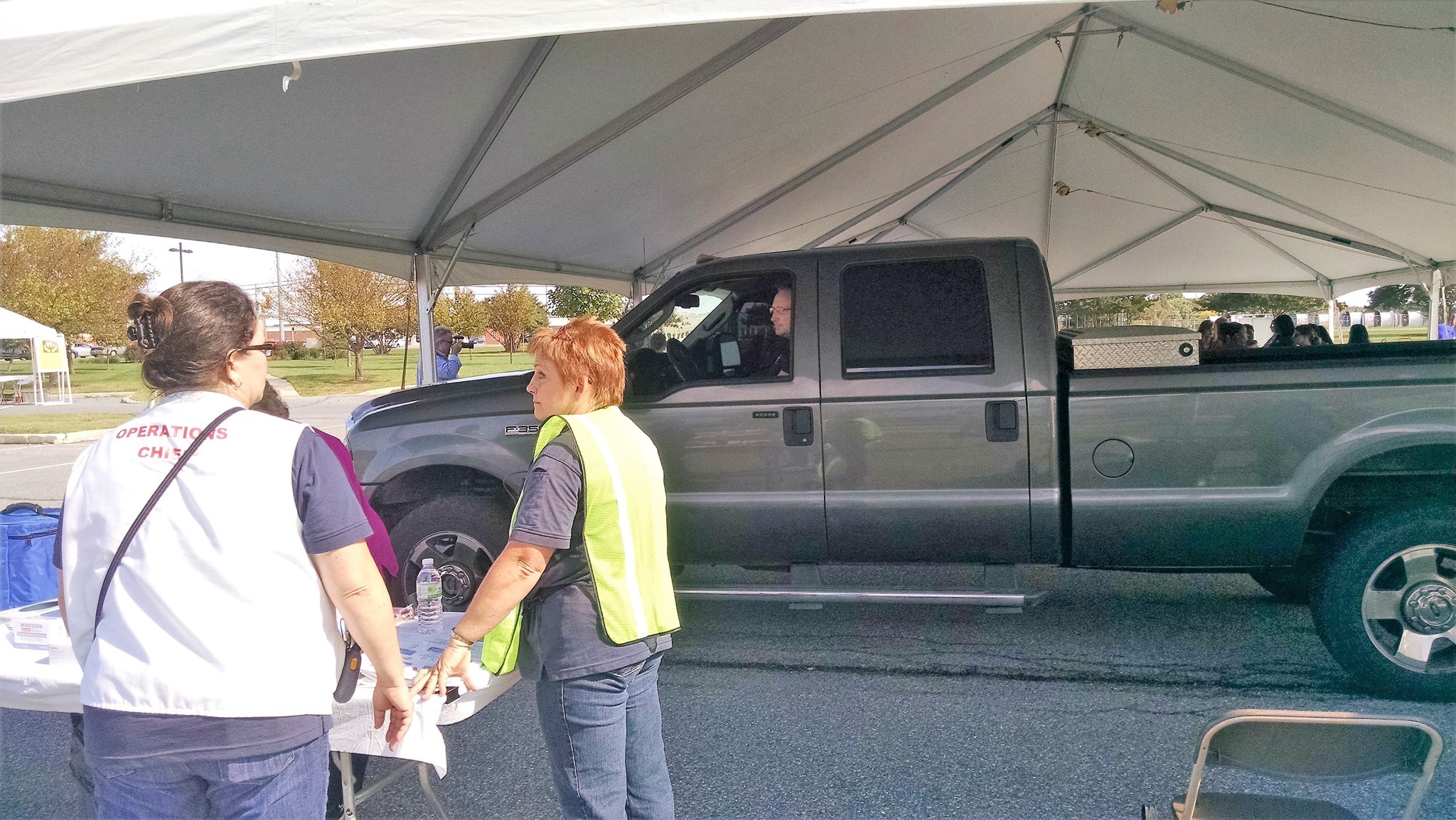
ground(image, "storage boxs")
xmin=20 ymin=388 xmax=50 ymax=402
xmin=1058 ymin=323 xmax=1199 ymax=372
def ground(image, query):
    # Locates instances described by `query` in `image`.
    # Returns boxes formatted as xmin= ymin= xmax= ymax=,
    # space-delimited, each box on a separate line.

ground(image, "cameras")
xmin=451 ymin=336 xmax=473 ymax=349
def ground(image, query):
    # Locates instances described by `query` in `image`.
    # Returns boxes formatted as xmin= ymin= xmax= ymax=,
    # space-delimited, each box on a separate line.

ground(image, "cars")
xmin=1 ymin=345 xmax=31 ymax=361
xmin=363 ymin=334 xmax=401 ymax=349
xmin=90 ymin=342 xmax=126 ymax=357
xmin=350 ymin=335 xmax=357 ymax=344
xmin=470 ymin=337 xmax=485 ymax=344
xmin=65 ymin=342 xmax=93 ymax=359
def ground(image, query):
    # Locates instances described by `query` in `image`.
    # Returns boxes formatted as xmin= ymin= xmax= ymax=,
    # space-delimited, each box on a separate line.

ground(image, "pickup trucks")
xmin=341 ymin=236 xmax=1455 ymax=703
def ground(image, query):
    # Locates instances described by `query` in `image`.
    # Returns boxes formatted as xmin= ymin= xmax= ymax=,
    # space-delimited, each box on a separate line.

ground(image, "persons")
xmin=418 ymin=327 xmax=463 ymax=386
xmin=738 ymin=282 xmax=794 ymax=379
xmin=412 ymin=316 xmax=677 ymax=820
xmin=1196 ymin=313 xmax=1456 ymax=347
xmin=53 ymin=281 xmax=413 ymax=820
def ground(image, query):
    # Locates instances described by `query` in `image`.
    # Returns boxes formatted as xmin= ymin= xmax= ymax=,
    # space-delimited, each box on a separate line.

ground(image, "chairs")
xmin=1140 ymin=706 xmax=1449 ymax=820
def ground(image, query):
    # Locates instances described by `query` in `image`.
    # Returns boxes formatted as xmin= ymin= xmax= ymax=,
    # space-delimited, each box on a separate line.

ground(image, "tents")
xmin=0 ymin=307 xmax=58 ymax=405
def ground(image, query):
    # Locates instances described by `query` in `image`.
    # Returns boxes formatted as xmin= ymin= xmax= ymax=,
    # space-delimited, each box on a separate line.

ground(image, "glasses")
xmin=442 ymin=339 xmax=453 ymax=346
xmin=237 ymin=341 xmax=277 ymax=357
xmin=769 ymin=306 xmax=791 ymax=314
xmin=551 ymin=326 xmax=592 ymax=385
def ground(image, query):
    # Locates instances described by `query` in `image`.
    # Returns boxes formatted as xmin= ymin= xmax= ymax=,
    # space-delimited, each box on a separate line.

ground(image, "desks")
xmin=0 ymin=598 xmax=522 ymax=820
xmin=0 ymin=375 xmax=35 ymax=405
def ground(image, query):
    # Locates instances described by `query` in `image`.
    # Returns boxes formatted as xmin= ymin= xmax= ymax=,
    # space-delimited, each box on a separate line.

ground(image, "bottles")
xmin=416 ymin=558 xmax=444 ymax=634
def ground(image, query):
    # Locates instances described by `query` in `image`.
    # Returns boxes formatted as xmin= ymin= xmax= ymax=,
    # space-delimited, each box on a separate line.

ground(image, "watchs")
xmin=449 ymin=627 xmax=474 ymax=646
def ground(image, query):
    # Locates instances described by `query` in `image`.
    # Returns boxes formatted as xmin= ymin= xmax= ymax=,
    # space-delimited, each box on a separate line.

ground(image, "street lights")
xmin=168 ymin=243 xmax=194 ymax=283
